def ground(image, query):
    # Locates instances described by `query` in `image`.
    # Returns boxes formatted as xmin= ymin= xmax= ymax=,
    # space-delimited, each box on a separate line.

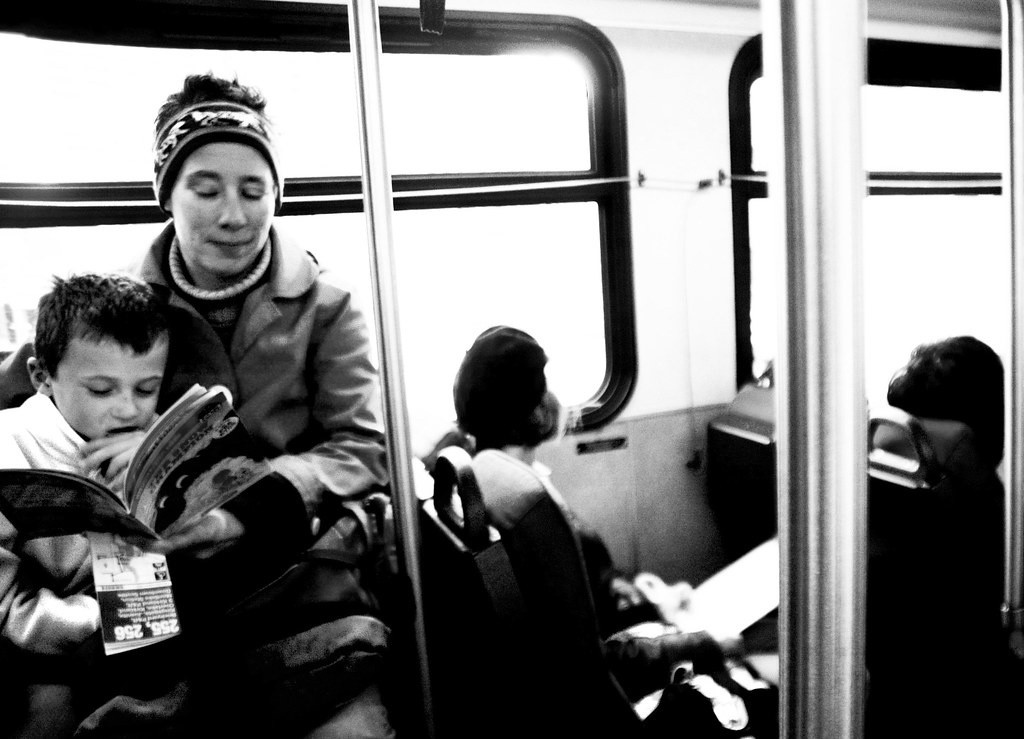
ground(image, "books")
xmin=0 ymin=383 xmax=275 ymax=540
xmin=87 ymin=532 xmax=181 ymax=656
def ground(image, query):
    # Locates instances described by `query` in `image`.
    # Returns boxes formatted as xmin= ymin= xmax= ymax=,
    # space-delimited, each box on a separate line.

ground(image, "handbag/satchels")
xmin=633 ymin=646 xmax=779 ymax=739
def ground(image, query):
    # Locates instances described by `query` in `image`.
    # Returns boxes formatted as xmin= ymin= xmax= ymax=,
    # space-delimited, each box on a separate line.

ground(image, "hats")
xmin=453 ymin=327 xmax=547 ymax=436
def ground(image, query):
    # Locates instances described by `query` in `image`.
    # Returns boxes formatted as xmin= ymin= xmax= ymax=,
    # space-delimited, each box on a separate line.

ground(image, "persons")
xmin=891 ymin=337 xmax=1005 ymax=477
xmin=0 ymin=274 xmax=175 ymax=739
xmin=453 ymin=325 xmax=779 ymax=739
xmin=138 ymin=75 xmax=405 ymax=739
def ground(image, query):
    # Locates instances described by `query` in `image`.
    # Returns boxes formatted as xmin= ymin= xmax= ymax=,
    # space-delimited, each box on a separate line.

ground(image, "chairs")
xmin=701 ymin=377 xmax=1024 ymax=739
xmin=371 ymin=446 xmax=652 ymax=739
